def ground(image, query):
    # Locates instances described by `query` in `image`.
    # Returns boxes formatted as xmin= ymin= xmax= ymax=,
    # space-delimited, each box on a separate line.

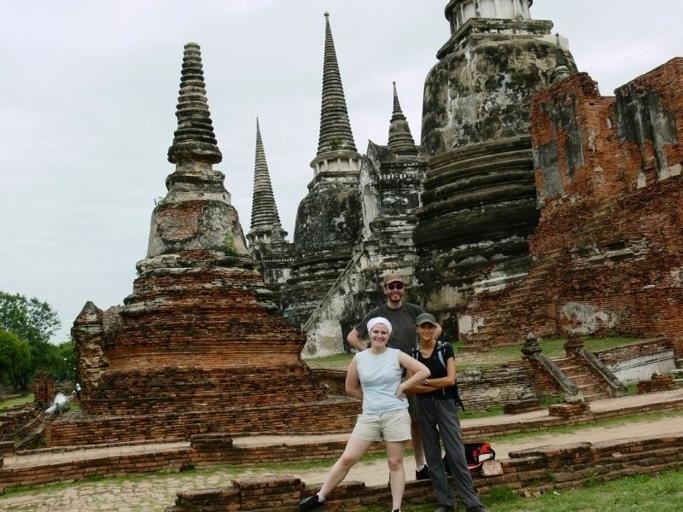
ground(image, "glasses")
xmin=388 ymin=284 xmax=403 ymax=290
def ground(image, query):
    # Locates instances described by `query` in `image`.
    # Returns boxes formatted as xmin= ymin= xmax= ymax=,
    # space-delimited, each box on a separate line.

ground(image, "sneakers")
xmin=431 ymin=504 xmax=450 ymax=512
xmin=298 ymin=489 xmax=325 ymax=512
xmin=414 ymin=466 xmax=430 ymax=479
xmin=465 ymin=504 xmax=490 ymax=512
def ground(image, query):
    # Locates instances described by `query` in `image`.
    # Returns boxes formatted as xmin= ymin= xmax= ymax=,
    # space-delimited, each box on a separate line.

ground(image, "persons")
xmin=297 ymin=317 xmax=432 ymax=512
xmin=43 ymin=391 xmax=69 ymax=420
xmin=72 ymin=380 xmax=82 ymax=399
xmin=406 ymin=313 xmax=487 ymax=512
xmin=347 ymin=273 xmax=442 ymax=480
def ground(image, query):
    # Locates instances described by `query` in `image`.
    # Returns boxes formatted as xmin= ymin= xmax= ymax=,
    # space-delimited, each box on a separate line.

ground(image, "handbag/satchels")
xmin=443 ymin=442 xmax=495 ymax=474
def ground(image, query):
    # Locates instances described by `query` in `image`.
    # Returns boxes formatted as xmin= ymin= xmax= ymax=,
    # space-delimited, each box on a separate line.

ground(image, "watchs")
xmin=424 ymin=380 xmax=429 ymax=386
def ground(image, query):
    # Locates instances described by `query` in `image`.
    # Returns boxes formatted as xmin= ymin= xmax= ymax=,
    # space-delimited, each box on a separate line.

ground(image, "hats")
xmin=416 ymin=313 xmax=437 ymax=327
xmin=384 ymin=270 xmax=405 ymax=286
xmin=366 ymin=317 xmax=392 ymax=336
